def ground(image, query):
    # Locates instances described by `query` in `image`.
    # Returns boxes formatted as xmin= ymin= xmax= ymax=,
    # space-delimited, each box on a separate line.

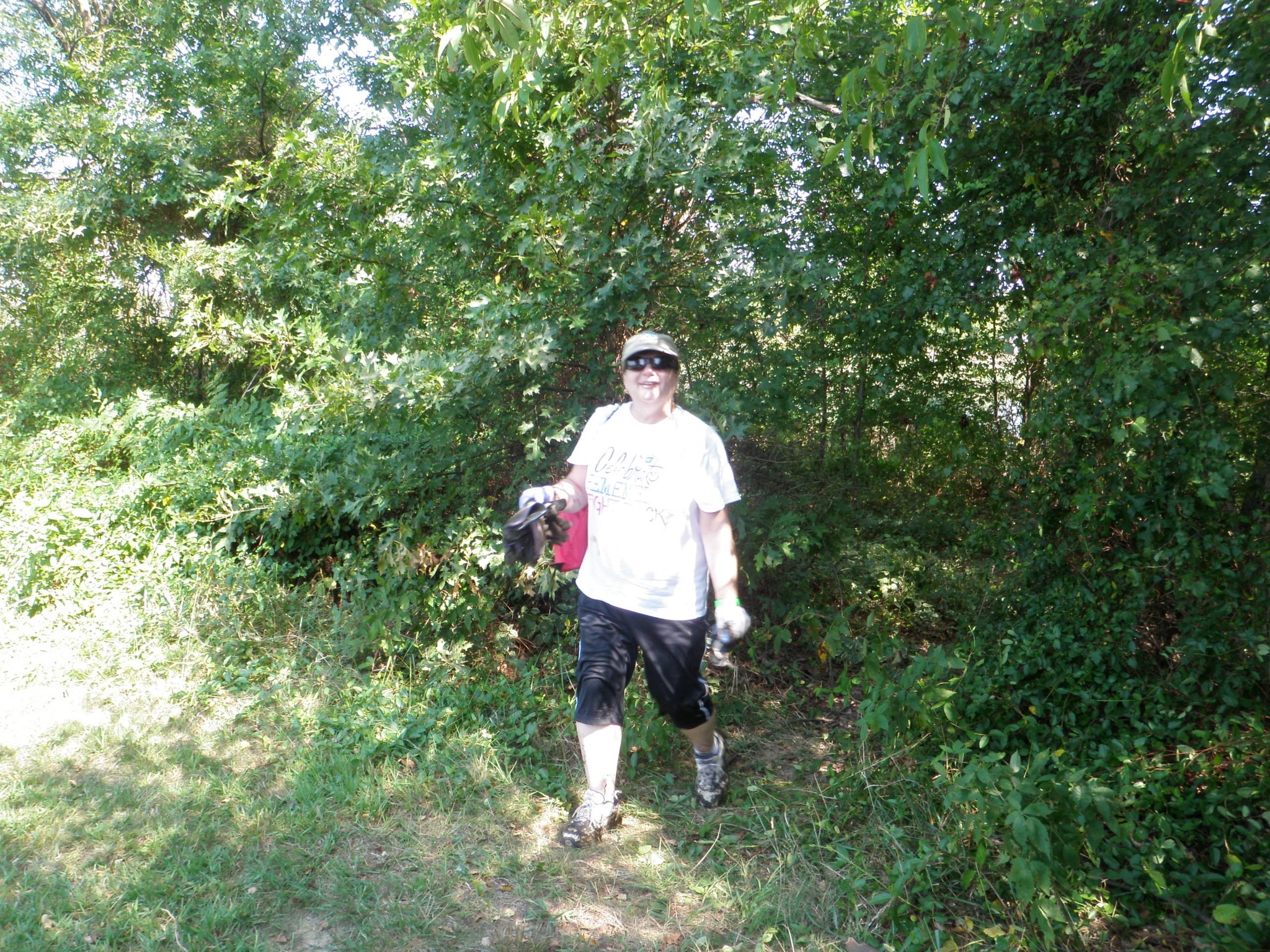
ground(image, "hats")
xmin=621 ymin=330 xmax=679 ymax=359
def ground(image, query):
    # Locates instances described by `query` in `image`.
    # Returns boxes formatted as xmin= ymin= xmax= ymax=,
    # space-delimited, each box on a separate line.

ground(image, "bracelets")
xmin=715 ymin=598 xmax=742 ymax=609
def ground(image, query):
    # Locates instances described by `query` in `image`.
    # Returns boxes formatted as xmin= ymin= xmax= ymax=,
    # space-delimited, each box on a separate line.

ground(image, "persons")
xmin=518 ymin=333 xmax=753 ymax=848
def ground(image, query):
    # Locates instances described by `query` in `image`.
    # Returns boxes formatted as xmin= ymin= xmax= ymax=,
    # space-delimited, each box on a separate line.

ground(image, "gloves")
xmin=714 ymin=601 xmax=752 ymax=638
xmin=518 ymin=484 xmax=555 ymax=509
xmin=505 ymin=497 xmax=571 ymax=564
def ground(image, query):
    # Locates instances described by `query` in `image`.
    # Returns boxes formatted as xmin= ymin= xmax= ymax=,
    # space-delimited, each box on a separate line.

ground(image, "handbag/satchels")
xmin=550 ymin=477 xmax=588 ymax=572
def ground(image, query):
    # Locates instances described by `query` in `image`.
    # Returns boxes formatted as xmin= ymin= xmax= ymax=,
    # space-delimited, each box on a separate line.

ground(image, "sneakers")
xmin=563 ymin=789 xmax=621 ymax=848
xmin=694 ymin=731 xmax=729 ymax=808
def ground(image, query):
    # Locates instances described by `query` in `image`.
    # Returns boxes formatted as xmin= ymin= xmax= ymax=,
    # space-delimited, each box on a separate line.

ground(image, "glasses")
xmin=624 ymin=356 xmax=673 ymax=371
